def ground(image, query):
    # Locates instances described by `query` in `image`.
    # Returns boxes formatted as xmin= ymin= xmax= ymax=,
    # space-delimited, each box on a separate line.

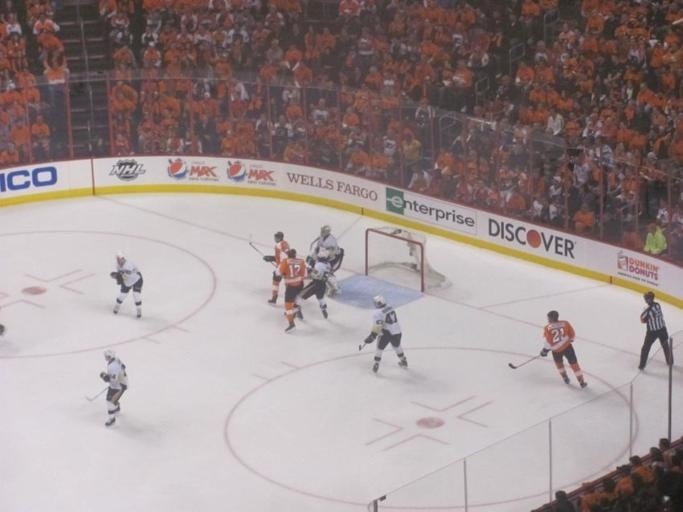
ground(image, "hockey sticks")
xmin=249 ymin=235 xmax=279 ymax=268
xmin=359 ymin=343 xmax=367 ymax=351
xmin=508 ymin=355 xmax=542 ymax=369
xmin=85 ymin=387 xmax=108 ymax=401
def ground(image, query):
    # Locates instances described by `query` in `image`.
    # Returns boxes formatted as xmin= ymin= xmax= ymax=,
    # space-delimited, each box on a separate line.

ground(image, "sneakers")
xmin=113 ymin=303 xmax=121 ymax=314
xmin=373 ymin=363 xmax=379 ymax=371
xmin=137 ymin=309 xmax=141 ymax=318
xmin=105 ymin=416 xmax=115 ymax=426
xmin=398 ymin=361 xmax=407 ymax=367
xmin=268 ymin=285 xmax=337 ymax=333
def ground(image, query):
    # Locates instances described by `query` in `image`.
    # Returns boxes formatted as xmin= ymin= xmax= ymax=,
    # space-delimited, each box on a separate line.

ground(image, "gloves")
xmin=540 ymin=348 xmax=549 ymax=357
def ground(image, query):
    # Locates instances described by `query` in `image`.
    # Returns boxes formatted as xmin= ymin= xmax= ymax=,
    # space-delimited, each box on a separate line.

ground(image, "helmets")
xmin=274 ymin=225 xmax=332 ymax=262
xmin=547 ymin=310 xmax=559 ymax=320
xmin=103 ymin=349 xmax=115 ymax=359
xmin=373 ymin=294 xmax=386 ymax=306
xmin=117 ymin=252 xmax=127 ymax=266
xmin=644 ymin=291 xmax=654 ymax=301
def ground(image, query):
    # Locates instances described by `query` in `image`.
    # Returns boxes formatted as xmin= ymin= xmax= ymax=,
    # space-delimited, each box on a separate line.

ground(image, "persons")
xmin=268 ymin=225 xmax=339 ymax=332
xmin=535 ymin=434 xmax=682 ymax=511
xmin=542 ymin=309 xmax=589 ymax=389
xmin=112 ymin=248 xmax=144 ymax=320
xmin=99 ymin=347 xmax=128 ymax=426
xmin=635 ymin=291 xmax=675 ymax=371
xmin=2 ymin=0 xmax=683 ymax=268
xmin=362 ymin=295 xmax=408 ymax=373
